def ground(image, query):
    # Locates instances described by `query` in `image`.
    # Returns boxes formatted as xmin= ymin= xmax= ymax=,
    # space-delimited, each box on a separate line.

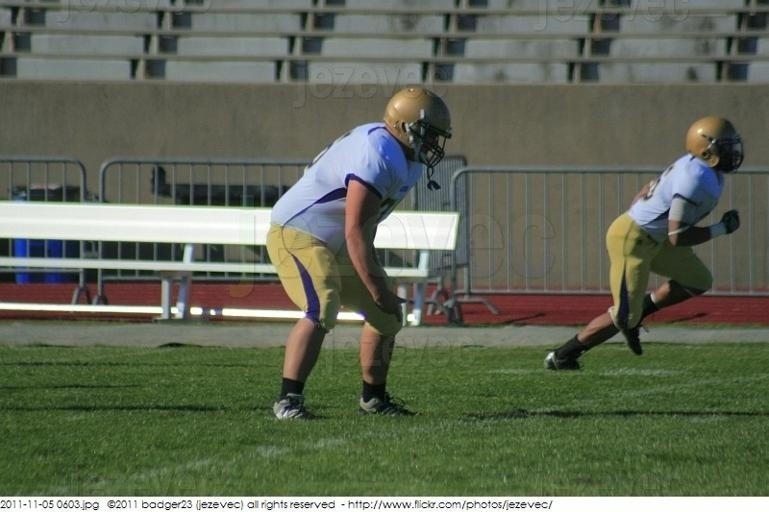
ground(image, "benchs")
xmin=0 ymin=201 xmax=461 ymax=332
xmin=0 ymin=2 xmax=768 ymax=85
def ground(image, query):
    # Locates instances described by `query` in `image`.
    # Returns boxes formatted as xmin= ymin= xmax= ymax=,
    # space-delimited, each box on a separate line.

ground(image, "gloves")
xmin=719 ymin=209 xmax=740 ymax=234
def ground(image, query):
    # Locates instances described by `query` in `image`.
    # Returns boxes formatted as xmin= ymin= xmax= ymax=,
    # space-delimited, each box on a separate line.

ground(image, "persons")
xmin=265 ymin=86 xmax=452 ymax=421
xmin=542 ymin=114 xmax=744 ymax=372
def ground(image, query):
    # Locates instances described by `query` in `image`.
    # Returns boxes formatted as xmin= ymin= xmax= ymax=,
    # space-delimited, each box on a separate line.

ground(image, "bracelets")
xmin=708 ymin=221 xmax=727 ymax=239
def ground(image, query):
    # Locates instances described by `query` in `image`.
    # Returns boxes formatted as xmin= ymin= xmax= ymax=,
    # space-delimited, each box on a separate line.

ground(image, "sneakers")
xmin=358 ymin=392 xmax=420 ymax=416
xmin=544 ymin=351 xmax=580 ymax=371
xmin=621 ymin=324 xmax=644 ymax=355
xmin=273 ymin=393 xmax=315 ymax=420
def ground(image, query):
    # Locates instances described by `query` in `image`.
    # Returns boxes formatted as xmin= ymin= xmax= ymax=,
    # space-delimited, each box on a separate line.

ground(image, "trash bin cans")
xmin=6 ymin=184 xmax=89 ymax=284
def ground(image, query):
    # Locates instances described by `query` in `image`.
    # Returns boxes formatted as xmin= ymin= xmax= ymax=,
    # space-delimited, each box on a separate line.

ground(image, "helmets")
xmin=686 ymin=116 xmax=740 ymax=168
xmin=384 ymin=87 xmax=452 ymax=149
